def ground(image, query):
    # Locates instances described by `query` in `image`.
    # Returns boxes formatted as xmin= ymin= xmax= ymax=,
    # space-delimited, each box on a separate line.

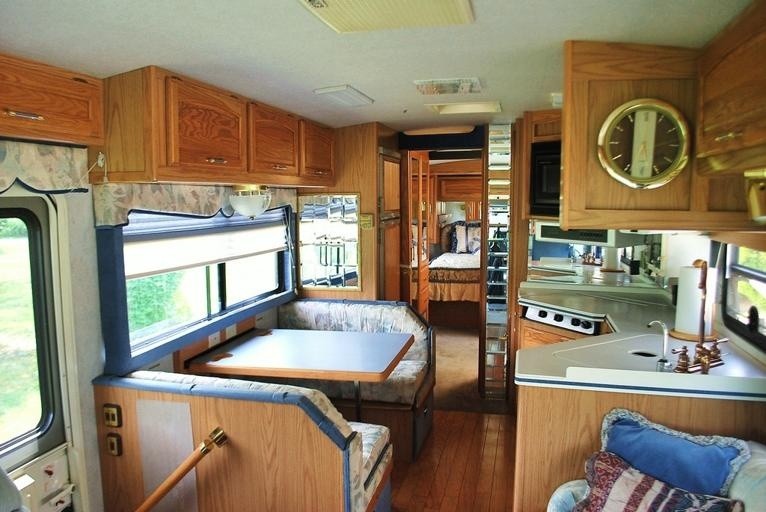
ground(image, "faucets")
xmin=646 ymin=319 xmax=674 ymax=373
xmin=568 ymin=244 xmax=585 ymax=260
xmin=694 ymin=261 xmax=710 ymax=373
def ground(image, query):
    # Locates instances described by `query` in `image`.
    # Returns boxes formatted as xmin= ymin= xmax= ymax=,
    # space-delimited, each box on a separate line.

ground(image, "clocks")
xmin=594 ymin=93 xmax=696 ymax=194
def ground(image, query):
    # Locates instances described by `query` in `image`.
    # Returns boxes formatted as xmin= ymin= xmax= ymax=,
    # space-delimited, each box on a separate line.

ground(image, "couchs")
xmin=544 ymin=433 xmax=765 ymax=512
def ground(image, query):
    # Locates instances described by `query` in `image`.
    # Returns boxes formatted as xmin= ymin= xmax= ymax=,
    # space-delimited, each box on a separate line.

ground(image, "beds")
xmin=429 ymin=216 xmax=486 ymax=306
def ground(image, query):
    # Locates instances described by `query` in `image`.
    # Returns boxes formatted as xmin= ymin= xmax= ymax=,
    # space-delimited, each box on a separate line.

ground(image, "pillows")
xmin=564 ymin=443 xmax=747 ymax=512
xmin=465 ymin=221 xmax=484 ymax=255
xmin=447 ymin=220 xmax=469 ymax=254
xmin=583 ymin=399 xmax=751 ymax=508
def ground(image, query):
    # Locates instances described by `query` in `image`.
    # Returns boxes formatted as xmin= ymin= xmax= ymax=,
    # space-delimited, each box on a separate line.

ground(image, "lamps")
xmin=227 ymin=183 xmax=273 ymax=223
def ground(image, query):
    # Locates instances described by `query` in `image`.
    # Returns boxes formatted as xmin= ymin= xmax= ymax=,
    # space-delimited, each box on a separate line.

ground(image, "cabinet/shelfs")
xmin=246 ymin=97 xmax=300 ymax=191
xmin=515 ymin=317 xmax=596 ymax=353
xmin=296 ymin=113 xmax=339 ymax=192
xmin=82 ymin=64 xmax=250 ymax=190
xmin=336 ymin=120 xmax=404 ymax=302
xmin=518 ymin=103 xmax=564 ymax=224
xmin=406 ymin=150 xmax=431 ymax=328
xmin=693 ymin=0 xmax=766 ymax=174
xmin=0 ymin=52 xmax=105 ymax=151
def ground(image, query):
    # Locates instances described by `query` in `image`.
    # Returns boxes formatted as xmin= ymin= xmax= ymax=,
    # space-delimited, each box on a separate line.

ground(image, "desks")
xmin=184 ymin=323 xmax=421 ymax=429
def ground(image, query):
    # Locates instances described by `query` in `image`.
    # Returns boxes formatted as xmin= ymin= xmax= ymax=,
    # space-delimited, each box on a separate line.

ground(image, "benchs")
xmin=253 ymin=293 xmax=443 ymax=467
xmin=89 ymin=362 xmax=397 ymax=512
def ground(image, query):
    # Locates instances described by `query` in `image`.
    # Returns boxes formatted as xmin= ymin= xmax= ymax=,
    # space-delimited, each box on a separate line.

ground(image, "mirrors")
xmin=479 ymin=119 xmax=518 ymax=401
xmin=291 ymin=189 xmax=363 ymax=293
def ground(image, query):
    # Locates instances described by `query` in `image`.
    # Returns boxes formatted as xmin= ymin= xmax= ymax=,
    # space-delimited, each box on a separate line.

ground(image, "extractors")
xmin=533 ymin=222 xmax=648 ymax=248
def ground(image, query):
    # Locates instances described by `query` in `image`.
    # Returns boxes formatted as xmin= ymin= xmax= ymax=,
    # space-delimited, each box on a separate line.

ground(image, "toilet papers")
xmin=607 ymin=248 xmax=622 ymax=270
xmin=674 ymin=266 xmax=719 ymax=337
